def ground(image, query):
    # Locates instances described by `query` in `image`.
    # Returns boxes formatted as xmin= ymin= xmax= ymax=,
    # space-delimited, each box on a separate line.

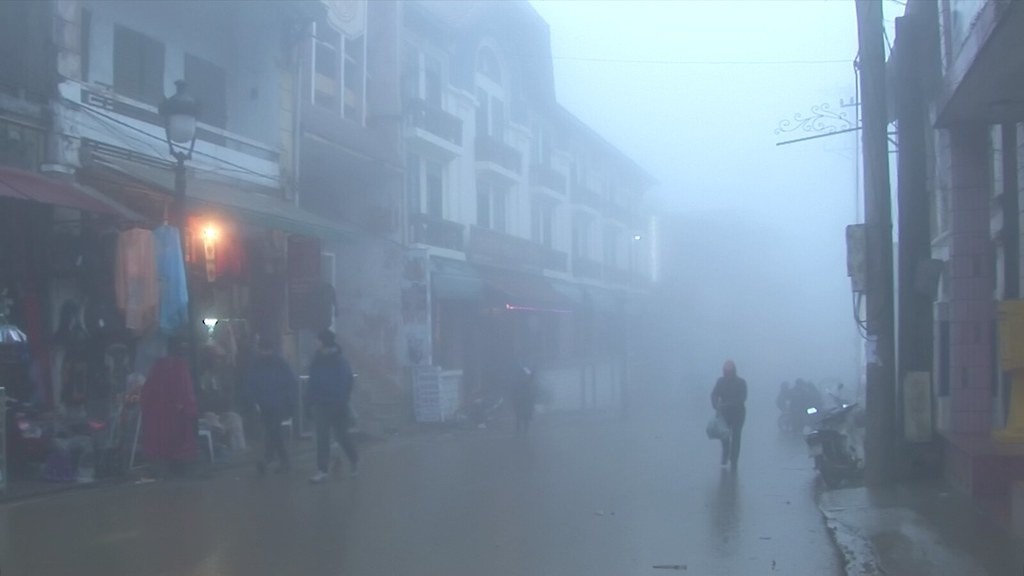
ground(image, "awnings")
xmin=0 ymin=162 xmax=143 ymax=219
xmin=484 ymin=269 xmax=574 ymax=313
xmin=87 ymin=151 xmax=356 ymax=235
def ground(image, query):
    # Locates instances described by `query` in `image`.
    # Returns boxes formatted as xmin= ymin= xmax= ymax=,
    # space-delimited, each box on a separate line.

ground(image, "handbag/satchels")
xmin=705 ymin=411 xmax=731 ymax=440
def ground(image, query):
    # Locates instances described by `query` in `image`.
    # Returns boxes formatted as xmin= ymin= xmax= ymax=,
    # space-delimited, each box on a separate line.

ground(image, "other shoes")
xmin=310 ymin=471 xmax=330 ymax=484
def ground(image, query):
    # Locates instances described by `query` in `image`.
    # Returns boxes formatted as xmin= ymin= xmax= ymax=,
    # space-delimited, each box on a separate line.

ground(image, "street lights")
xmin=156 ymin=77 xmax=216 ymax=409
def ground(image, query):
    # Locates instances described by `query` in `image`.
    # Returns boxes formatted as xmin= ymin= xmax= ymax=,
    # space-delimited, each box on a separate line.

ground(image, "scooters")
xmin=798 ymin=399 xmax=861 ymax=489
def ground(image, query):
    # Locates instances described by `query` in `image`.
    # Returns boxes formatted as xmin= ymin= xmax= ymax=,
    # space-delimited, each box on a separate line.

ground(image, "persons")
xmin=777 ymin=378 xmax=821 ymax=433
xmin=706 ymin=360 xmax=748 ymax=468
xmin=245 ymin=333 xmax=298 ymax=473
xmin=308 ymin=329 xmax=359 ymax=484
xmin=141 ymin=338 xmax=199 ymax=480
